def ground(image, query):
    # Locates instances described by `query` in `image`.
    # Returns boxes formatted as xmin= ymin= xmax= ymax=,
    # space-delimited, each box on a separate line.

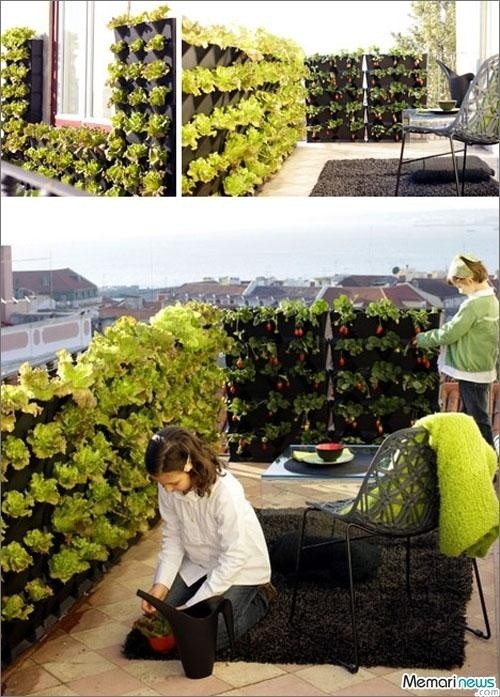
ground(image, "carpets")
xmin=308 ymin=154 xmax=498 ymax=197
xmin=117 ymin=507 xmax=475 ymax=674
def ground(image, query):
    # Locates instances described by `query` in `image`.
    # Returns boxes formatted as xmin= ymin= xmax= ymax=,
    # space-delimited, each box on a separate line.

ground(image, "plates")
xmin=430 ymin=107 xmax=461 ymax=114
xmin=291 ymin=446 xmax=355 ymax=465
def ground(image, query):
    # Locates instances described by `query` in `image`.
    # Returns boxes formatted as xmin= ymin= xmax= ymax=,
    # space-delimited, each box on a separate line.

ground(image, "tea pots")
xmin=435 ymin=58 xmax=474 ymax=107
xmin=135 ymin=587 xmax=237 ymax=679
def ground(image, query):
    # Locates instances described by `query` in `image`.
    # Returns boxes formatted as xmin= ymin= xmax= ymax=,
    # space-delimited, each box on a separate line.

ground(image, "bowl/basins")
xmin=315 ymin=443 xmax=344 ymax=462
xmin=439 ymin=100 xmax=457 ymax=111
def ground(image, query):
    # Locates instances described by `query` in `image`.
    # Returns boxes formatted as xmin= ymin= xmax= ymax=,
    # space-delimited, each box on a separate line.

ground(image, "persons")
xmin=141 ymin=427 xmax=280 ymax=649
xmin=413 ymin=254 xmax=500 ymax=483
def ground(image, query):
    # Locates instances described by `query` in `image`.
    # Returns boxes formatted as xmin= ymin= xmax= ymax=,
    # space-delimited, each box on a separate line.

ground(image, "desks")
xmin=260 ymin=440 xmax=404 ymax=540
xmin=397 ymin=105 xmax=473 ymax=193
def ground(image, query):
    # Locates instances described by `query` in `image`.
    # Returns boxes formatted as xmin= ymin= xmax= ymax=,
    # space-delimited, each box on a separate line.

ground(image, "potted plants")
xmin=133 ymin=604 xmax=178 ymax=653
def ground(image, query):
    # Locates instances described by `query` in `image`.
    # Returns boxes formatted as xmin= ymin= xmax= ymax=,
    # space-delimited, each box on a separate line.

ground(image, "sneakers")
xmin=260 ymin=582 xmax=278 ymax=603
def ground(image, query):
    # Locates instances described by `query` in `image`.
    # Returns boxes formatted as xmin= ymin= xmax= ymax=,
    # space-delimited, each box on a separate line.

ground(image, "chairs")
xmin=393 ymin=54 xmax=500 ymax=195
xmin=286 ymin=420 xmax=493 ymax=673
